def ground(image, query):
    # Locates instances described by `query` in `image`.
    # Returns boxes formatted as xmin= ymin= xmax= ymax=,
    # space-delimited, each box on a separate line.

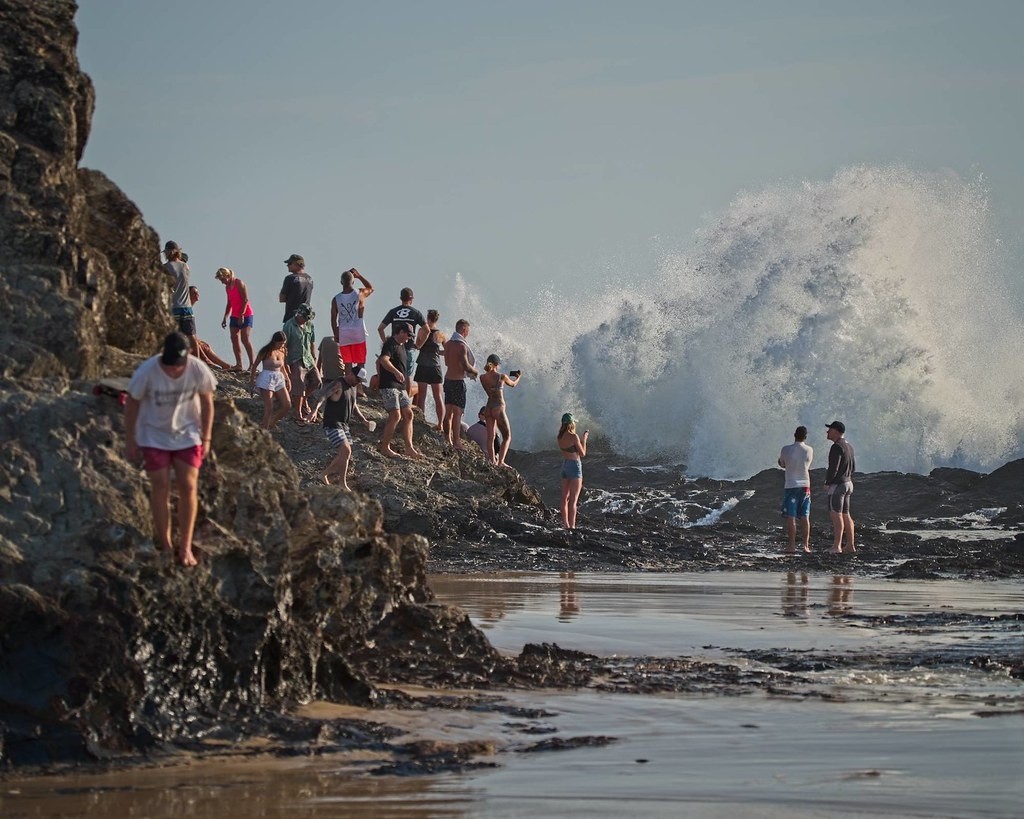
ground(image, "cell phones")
xmin=585 ymin=430 xmax=589 ymax=432
xmin=510 ymin=371 xmax=520 ymax=376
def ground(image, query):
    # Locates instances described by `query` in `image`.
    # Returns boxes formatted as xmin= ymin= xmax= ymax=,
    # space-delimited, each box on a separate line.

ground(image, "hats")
xmin=161 ymin=331 xmax=190 ymax=366
xmin=284 ymin=254 xmax=304 ymax=267
xmin=825 ymin=421 xmax=845 ymax=433
xmin=562 ymin=413 xmax=579 ymax=424
xmin=162 ymin=241 xmax=177 ymax=252
xmin=488 ymin=354 xmax=501 ymax=366
xmin=353 ymin=366 xmax=368 ymax=383
xmin=398 ymin=323 xmax=416 ymax=336
xmin=293 ymin=303 xmax=315 ymax=320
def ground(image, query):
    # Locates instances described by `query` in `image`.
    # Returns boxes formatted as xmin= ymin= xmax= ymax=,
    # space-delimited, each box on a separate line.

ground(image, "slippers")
xmin=302 ymin=405 xmax=312 ymax=415
xmin=290 ymin=416 xmax=307 ymax=426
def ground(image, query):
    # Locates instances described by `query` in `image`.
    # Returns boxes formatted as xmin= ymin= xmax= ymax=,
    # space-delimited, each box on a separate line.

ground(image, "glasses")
xmin=406 ymin=333 xmax=412 ymax=337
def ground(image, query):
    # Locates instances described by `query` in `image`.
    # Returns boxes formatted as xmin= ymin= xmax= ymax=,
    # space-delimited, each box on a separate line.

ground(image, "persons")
xmin=283 ymin=302 xmax=322 ymax=420
xmin=479 ymin=355 xmax=522 ymax=469
xmin=331 ymin=268 xmax=374 ymax=396
xmin=317 ymin=333 xmax=346 ymax=383
xmin=307 ymin=367 xmax=368 ymax=491
xmin=824 ymin=421 xmax=857 ymax=552
xmin=250 ymin=331 xmax=292 ymax=432
xmin=279 ymin=254 xmax=314 ymax=323
xmin=126 ymin=332 xmax=219 ymax=565
xmin=161 ymin=240 xmax=232 ymax=368
xmin=558 ymin=413 xmax=589 ymax=529
xmin=379 ymin=322 xmax=419 ymax=459
xmin=215 ymin=267 xmax=255 ymax=372
xmin=416 ymin=309 xmax=447 ymax=429
xmin=443 ymin=319 xmax=478 ymax=452
xmin=466 ymin=406 xmax=500 ymax=463
xmin=778 ymin=426 xmax=814 ymax=553
xmin=377 ymin=287 xmax=430 ymax=396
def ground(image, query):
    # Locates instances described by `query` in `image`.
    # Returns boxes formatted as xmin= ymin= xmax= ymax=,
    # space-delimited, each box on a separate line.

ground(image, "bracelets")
xmin=201 ymin=437 xmax=212 ymax=441
